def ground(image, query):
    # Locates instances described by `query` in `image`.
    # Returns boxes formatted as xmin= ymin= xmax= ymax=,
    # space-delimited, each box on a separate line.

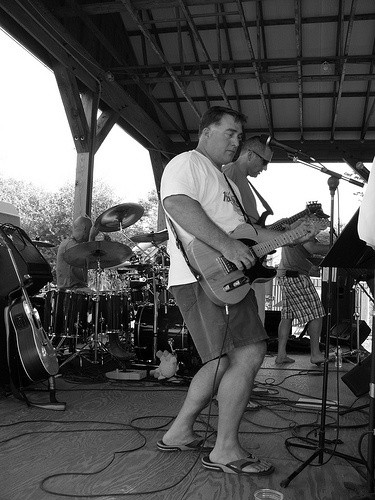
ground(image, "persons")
xmin=358 ymin=157 xmax=375 ymax=249
xmin=221 ymin=138 xmax=304 ymax=410
xmin=54 ymin=217 xmax=111 ymax=335
xmin=156 ymin=105 xmax=318 ymax=476
xmin=275 ymin=215 xmax=334 ymax=366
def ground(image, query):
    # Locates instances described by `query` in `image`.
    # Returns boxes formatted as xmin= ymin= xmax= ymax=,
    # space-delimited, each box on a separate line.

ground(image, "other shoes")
xmin=275 ymin=356 xmax=295 ymax=364
xmin=310 ymin=353 xmax=335 ymax=364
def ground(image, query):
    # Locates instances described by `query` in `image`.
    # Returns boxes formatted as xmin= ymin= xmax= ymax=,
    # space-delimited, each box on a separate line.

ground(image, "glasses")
xmin=249 ymin=150 xmax=269 ymax=165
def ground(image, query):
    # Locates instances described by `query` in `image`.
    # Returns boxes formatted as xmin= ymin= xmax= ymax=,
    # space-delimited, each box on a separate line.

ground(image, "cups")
xmin=254 ymin=488 xmax=284 ymax=500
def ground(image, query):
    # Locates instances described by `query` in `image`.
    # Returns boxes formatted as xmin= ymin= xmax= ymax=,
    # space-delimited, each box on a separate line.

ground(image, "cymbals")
xmin=93 ymin=202 xmax=144 ymax=233
xmin=128 ymin=231 xmax=169 ymax=243
xmin=32 ymin=240 xmax=56 ymax=248
xmin=63 ymin=239 xmax=133 ymax=270
xmin=123 ymin=264 xmax=157 ymax=269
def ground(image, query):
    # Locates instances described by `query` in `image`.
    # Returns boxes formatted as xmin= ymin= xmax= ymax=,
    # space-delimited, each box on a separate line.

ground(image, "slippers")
xmin=201 ymin=450 xmax=275 ymax=476
xmin=157 ymin=433 xmax=216 ymax=451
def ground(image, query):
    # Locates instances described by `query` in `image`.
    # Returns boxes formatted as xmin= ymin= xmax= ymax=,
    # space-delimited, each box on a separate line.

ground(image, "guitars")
xmin=0 ymin=225 xmax=59 ymax=384
xmin=186 ymin=215 xmax=331 ymax=308
xmin=254 ymin=200 xmax=322 ymax=255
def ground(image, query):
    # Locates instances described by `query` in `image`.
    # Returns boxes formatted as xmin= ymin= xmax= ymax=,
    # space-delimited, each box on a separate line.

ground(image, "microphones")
xmin=259 ymin=134 xmax=317 ymax=162
xmin=355 ymin=161 xmax=370 ymax=174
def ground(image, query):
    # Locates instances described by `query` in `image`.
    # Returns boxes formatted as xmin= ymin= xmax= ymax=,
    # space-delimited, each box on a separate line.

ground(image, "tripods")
xmin=59 ymin=259 xmax=127 ymax=372
xmin=279 ymin=154 xmax=368 ymax=488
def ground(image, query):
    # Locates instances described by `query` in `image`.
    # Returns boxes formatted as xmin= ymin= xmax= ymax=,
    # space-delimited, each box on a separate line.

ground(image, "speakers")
xmin=321 ymin=280 xmax=355 ymax=347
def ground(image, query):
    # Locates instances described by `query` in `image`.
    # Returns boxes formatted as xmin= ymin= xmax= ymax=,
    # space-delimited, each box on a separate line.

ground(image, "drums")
xmin=124 ymin=277 xmax=161 ymax=304
xmin=92 ymin=289 xmax=126 ymax=335
xmin=130 ymin=302 xmax=198 ymax=371
xmin=45 ymin=288 xmax=89 ymax=339
xmin=150 ymin=267 xmax=168 ymax=289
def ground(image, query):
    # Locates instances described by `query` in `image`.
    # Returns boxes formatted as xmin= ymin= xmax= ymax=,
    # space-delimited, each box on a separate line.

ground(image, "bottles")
xmin=334 ymin=346 xmax=343 ymax=368
